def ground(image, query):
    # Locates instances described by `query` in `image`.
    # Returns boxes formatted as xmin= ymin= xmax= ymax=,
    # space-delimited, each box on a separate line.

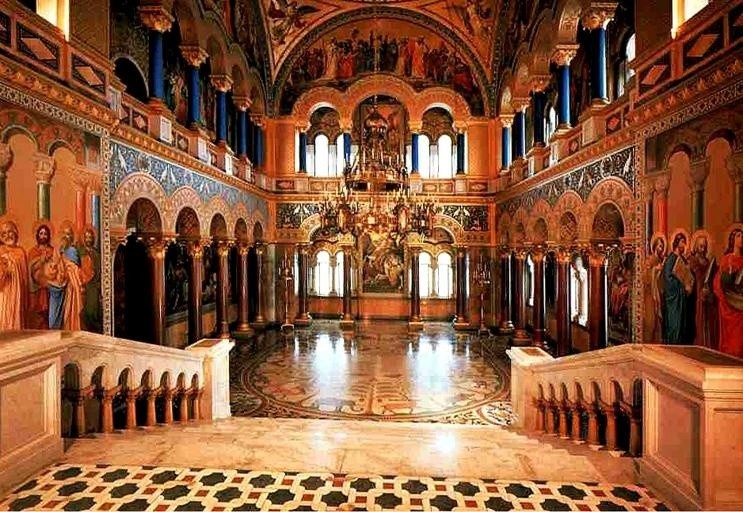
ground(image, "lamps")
xmin=320 ymin=0 xmax=437 ymax=237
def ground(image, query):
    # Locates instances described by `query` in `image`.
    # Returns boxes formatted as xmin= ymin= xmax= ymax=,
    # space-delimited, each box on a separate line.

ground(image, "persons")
xmin=0 ymin=220 xmax=104 ymax=333
xmin=361 ymin=102 xmax=391 ymax=151
xmin=291 ymin=27 xmax=482 ymax=107
xmin=642 ymin=224 xmax=742 ymax=360
